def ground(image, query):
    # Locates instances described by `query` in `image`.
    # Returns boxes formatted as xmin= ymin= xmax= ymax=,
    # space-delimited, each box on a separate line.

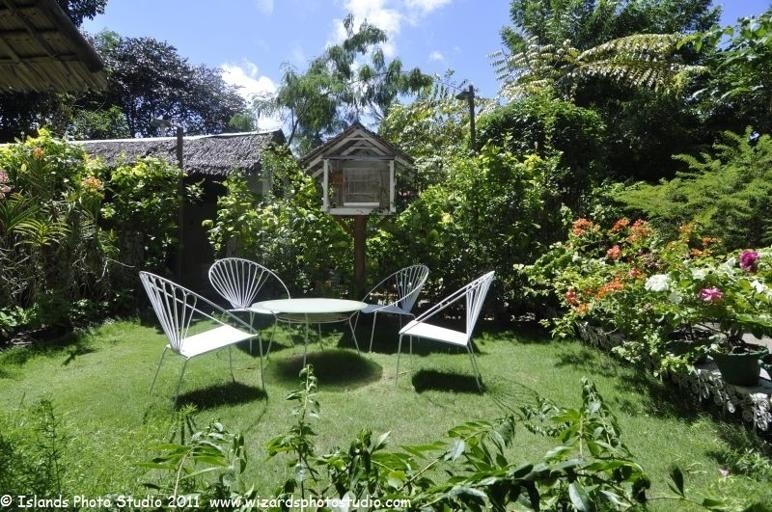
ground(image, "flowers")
xmin=641 ymin=242 xmax=772 ymax=378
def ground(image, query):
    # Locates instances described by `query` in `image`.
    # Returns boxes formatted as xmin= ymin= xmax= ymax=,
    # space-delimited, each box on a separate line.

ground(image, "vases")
xmin=711 ymin=344 xmax=768 ymax=388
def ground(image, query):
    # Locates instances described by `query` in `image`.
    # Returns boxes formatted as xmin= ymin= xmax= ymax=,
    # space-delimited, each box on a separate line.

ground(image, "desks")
xmin=251 ymin=298 xmax=368 ymax=368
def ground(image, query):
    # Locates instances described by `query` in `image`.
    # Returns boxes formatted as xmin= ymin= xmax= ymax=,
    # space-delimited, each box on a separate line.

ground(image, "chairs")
xmin=349 ymin=264 xmax=430 ymax=354
xmin=207 ymin=256 xmax=295 ymax=362
xmin=395 ymin=270 xmax=496 ymax=394
xmin=138 ymin=270 xmax=266 ymax=409
